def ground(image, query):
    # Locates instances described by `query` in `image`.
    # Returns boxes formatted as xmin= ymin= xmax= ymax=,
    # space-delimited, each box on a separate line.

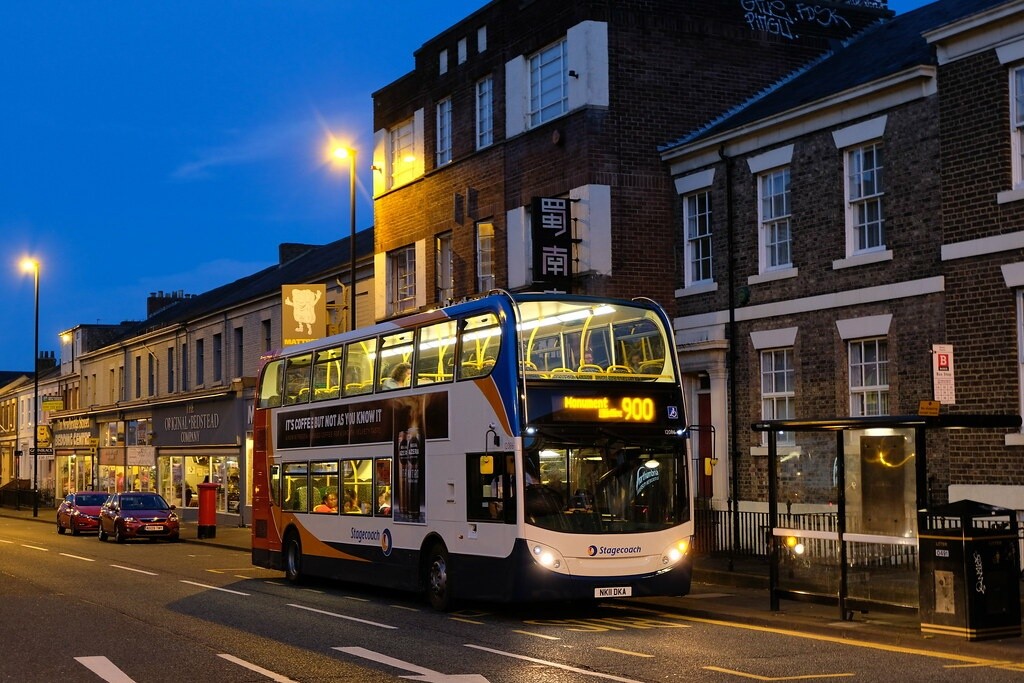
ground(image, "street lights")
xmin=329 ymin=139 xmax=356 ymax=330
xmin=19 ymin=254 xmax=40 ymax=518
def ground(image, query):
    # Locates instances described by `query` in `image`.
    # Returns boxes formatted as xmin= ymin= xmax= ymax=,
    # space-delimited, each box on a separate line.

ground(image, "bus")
xmin=250 ymin=286 xmax=719 ymax=616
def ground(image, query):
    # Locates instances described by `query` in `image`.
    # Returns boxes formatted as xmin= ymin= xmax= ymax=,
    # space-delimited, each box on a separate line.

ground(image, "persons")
xmin=315 ymin=489 xmax=392 ymax=516
xmin=573 ymin=351 xmax=595 ymax=372
xmin=382 ymin=362 xmax=411 ymax=390
xmin=488 ymin=472 xmax=532 ymax=520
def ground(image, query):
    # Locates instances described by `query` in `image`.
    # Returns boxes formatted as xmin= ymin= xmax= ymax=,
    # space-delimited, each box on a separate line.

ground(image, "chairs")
xmin=266 ymin=360 xmax=664 ymax=407
xmin=271 ymin=476 xmax=390 ymax=518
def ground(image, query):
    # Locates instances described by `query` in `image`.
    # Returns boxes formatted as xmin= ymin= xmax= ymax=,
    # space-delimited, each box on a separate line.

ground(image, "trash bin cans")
xmin=917 ymin=499 xmax=1022 ymax=642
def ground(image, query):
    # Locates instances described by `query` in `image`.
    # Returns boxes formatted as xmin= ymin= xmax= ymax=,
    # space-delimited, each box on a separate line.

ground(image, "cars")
xmin=57 ymin=492 xmax=112 ymax=537
xmin=97 ymin=490 xmax=180 ymax=543
